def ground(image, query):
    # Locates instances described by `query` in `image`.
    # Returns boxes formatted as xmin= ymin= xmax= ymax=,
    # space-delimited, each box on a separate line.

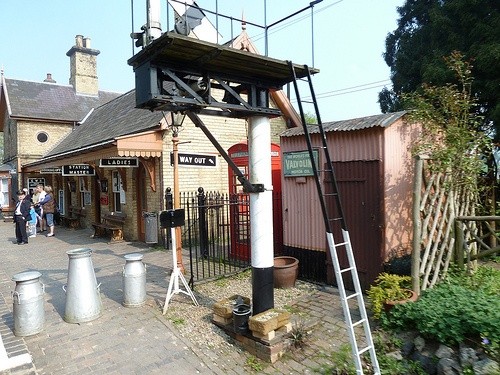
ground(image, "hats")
xmin=16 ymin=189 xmax=25 ymax=195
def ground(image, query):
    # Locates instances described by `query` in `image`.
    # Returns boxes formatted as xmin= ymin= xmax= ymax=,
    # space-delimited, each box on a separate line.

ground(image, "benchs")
xmin=63 ymin=206 xmax=82 ymax=230
xmin=91 ymin=210 xmax=127 ymax=244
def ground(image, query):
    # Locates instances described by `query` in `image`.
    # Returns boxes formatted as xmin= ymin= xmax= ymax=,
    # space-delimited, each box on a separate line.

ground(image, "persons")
xmin=21 ymin=184 xmax=55 ymax=238
xmin=12 ymin=190 xmax=33 ymax=245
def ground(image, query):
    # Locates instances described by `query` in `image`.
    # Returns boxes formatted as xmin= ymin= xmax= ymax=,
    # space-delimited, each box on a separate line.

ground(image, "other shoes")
xmin=13 ymin=241 xmax=18 ymax=244
xmin=18 ymin=242 xmax=27 ymax=245
xmin=37 ymin=230 xmax=42 ymax=233
xmin=29 ymin=235 xmax=36 ymax=237
xmin=43 ymin=230 xmax=47 ymax=231
xmin=46 ymin=233 xmax=54 ymax=236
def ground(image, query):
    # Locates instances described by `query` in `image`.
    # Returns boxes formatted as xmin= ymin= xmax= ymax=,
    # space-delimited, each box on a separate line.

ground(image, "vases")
xmin=274 ymin=256 xmax=299 ymax=288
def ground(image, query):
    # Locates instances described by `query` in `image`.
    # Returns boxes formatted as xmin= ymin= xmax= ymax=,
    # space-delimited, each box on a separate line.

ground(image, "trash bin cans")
xmin=144 ymin=212 xmax=157 ymax=243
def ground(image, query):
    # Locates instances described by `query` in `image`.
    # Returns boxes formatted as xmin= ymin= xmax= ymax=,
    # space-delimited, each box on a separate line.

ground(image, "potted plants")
xmin=367 ymin=272 xmax=417 ymax=321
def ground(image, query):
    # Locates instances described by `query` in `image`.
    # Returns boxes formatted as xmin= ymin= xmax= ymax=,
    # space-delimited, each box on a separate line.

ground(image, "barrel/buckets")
xmin=232 ymin=303 xmax=252 ymax=336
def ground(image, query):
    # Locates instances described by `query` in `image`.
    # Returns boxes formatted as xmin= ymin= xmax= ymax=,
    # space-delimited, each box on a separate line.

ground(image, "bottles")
xmin=61 ymin=248 xmax=105 ymax=324
xmin=11 ymin=271 xmax=47 ymax=337
xmin=122 ymin=253 xmax=147 ymax=308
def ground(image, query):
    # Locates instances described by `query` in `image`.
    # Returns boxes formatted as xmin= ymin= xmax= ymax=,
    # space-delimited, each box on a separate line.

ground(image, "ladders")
xmin=286 ymin=60 xmax=381 ymax=375
